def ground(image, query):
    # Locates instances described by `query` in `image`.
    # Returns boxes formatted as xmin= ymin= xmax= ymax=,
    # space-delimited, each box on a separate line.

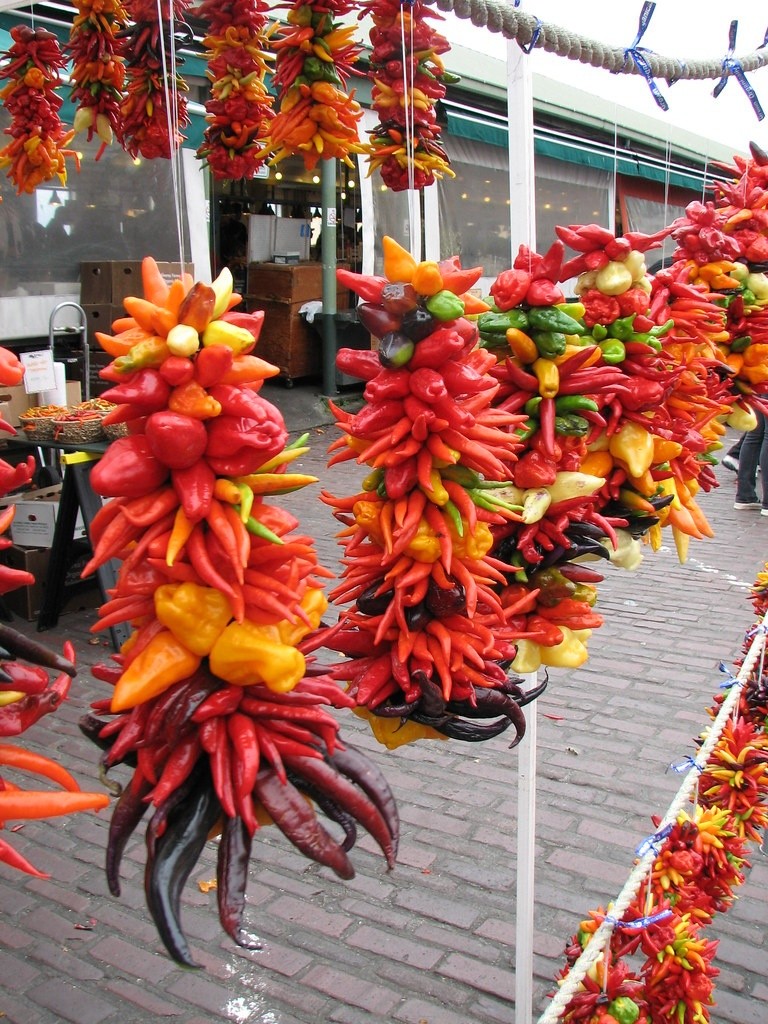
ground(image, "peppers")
xmin=0 ymin=0 xmax=461 ymax=187
xmin=0 ymin=142 xmax=768 ymax=961
xmin=546 ymin=567 xmax=768 ymax=1024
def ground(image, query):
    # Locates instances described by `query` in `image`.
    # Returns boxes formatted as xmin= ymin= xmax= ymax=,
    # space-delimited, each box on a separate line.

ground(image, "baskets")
xmin=18 ymin=410 xmax=128 ymax=444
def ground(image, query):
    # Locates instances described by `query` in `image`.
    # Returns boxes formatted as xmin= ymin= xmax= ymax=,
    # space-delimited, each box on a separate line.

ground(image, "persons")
xmin=221 ymin=203 xmax=247 ymax=256
xmin=722 ymin=393 xmax=768 ymax=518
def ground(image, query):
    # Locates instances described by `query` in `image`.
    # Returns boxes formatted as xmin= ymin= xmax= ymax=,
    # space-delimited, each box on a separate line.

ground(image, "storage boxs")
xmin=77 ymin=257 xmax=196 ymax=349
xmin=4 ymin=479 xmax=123 ymax=622
xmin=0 ymin=380 xmax=83 ymax=428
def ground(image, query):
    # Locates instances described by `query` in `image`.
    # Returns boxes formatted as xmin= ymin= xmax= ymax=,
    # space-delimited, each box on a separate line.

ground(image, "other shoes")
xmin=723 ymin=454 xmax=759 ymax=479
xmin=761 ymin=508 xmax=768 ymax=516
xmin=734 ymin=497 xmax=763 ymax=510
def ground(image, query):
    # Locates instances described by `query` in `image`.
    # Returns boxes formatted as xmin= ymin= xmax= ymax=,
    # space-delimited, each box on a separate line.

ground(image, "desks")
xmin=0 ymin=428 xmax=140 ymax=653
